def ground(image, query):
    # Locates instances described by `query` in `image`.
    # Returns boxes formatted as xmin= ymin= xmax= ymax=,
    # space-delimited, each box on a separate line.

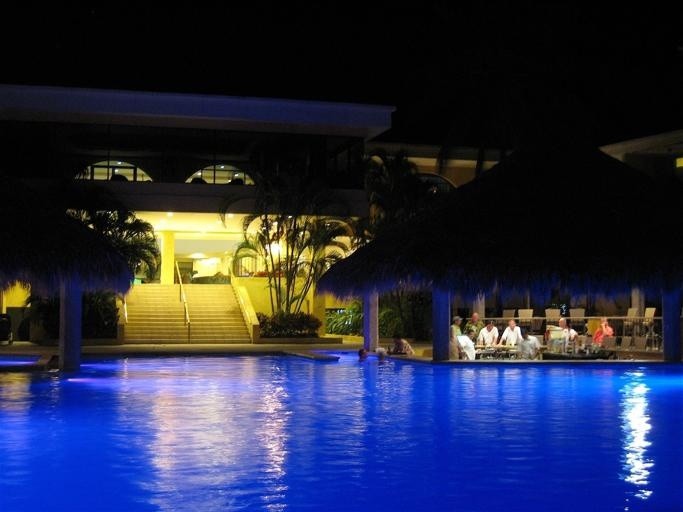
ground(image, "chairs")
xmin=490 ymin=307 xmax=657 ymax=350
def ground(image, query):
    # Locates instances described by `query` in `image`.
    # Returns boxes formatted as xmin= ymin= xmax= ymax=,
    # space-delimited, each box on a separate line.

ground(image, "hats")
xmin=453 ymin=316 xmax=464 ymax=322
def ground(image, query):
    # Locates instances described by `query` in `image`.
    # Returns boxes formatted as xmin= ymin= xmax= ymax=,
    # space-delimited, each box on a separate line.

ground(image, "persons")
xmin=478 ymin=320 xmax=499 ymax=346
xmin=358 ymin=349 xmax=370 ymax=362
xmin=451 ymin=312 xmax=484 ymax=360
xmin=377 ymin=351 xmax=385 ymax=360
xmin=592 ymin=318 xmax=613 ymax=348
xmin=387 ymin=334 xmax=415 ymax=355
xmin=550 ymin=317 xmax=576 ymax=345
xmin=498 ymin=318 xmax=523 ymax=346
xmin=517 ymin=327 xmax=540 ymax=360
